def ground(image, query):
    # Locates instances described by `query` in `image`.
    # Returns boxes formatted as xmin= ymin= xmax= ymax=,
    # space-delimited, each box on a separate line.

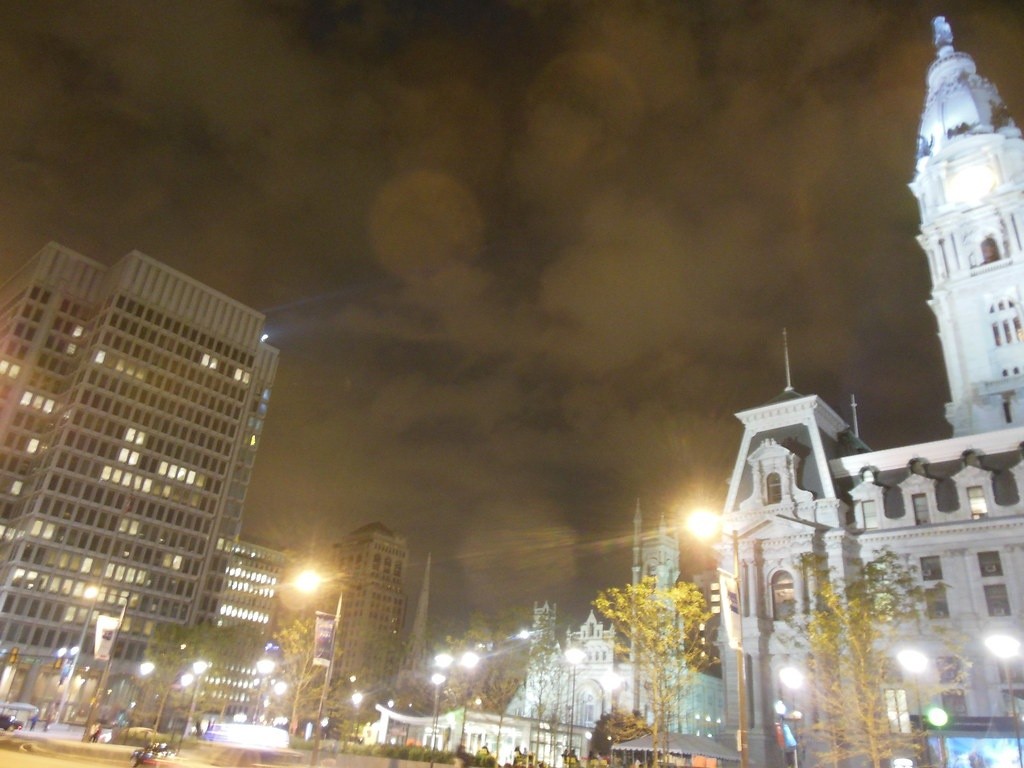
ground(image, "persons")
xmin=457 ymin=738 xmax=470 ymax=768
xmin=588 ymin=751 xmax=611 ymax=764
xmin=30 ymin=715 xmax=39 ymax=731
xmin=118 ymin=710 xmax=126 ymax=728
xmin=482 ymin=743 xmax=489 ymax=754
xmin=89 ymin=719 xmax=102 ymax=743
xmin=130 ymin=739 xmax=168 ymax=768
xmin=562 ymin=749 xmax=579 ymax=762
xmin=514 ymin=745 xmax=527 ymax=755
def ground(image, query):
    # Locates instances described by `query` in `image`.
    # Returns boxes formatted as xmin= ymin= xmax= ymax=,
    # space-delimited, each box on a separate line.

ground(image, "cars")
xmin=0 ymin=713 xmax=24 ymax=734
xmin=128 ymin=743 xmax=178 ymax=768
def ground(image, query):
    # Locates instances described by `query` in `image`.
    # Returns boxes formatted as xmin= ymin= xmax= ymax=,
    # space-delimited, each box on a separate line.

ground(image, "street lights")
xmin=138 ymin=660 xmax=192 ymax=743
xmin=927 ymin=707 xmax=949 ymax=768
xmin=181 ymin=659 xmax=209 ymax=743
xmin=986 ymin=633 xmax=1024 ymax=768
xmin=683 ymin=507 xmax=750 ymax=768
xmin=289 ymin=564 xmax=348 ymax=765
xmin=430 ymin=651 xmax=452 ymax=768
xmin=779 ymin=666 xmax=803 ymax=768
xmin=351 ymin=691 xmax=363 ymax=745
xmin=250 ymin=659 xmax=276 ymax=726
xmin=79 ymin=585 xmax=128 ymax=743
xmin=565 ymin=647 xmax=586 ymax=768
